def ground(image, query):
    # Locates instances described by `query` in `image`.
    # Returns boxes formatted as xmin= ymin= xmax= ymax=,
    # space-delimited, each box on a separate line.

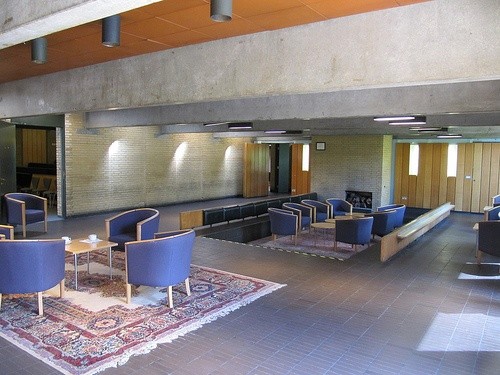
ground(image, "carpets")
xmin=0 ymin=249 xmax=288 ymax=375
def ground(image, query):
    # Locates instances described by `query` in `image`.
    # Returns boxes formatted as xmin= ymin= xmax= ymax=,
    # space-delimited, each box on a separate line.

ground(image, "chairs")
xmin=4 ymin=192 xmax=48 ymax=238
xmin=491 ymin=194 xmax=500 ymax=206
xmin=20 ymin=173 xmax=56 ymax=209
xmin=268 ymin=197 xmax=406 ymax=253
xmin=105 ymin=207 xmax=161 ymax=267
xmin=473 ymin=219 xmax=500 ymax=265
xmin=0 ymin=225 xmax=15 ymax=239
xmin=484 ymin=206 xmax=500 ymax=221
xmin=0 ymin=238 xmax=69 ymax=317
xmin=124 ymin=228 xmax=196 ymax=309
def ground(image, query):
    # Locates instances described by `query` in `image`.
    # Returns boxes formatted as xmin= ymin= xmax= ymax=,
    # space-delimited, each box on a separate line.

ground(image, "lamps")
xmin=101 ymin=14 xmax=121 ymax=48
xmin=210 ymin=0 xmax=232 ymax=22
xmin=373 ymin=115 xmax=462 ymax=138
xmin=31 ymin=37 xmax=49 ymax=64
xmin=199 ymin=121 xmax=307 ymax=136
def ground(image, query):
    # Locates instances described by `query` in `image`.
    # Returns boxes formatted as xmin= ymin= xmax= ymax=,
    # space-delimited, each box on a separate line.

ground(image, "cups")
xmin=88 ymin=234 xmax=96 ymax=241
xmin=62 ymin=237 xmax=71 ymax=244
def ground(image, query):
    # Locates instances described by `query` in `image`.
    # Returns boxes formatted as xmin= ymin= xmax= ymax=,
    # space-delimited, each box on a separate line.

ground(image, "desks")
xmin=324 ymin=218 xmax=336 ymax=223
xmin=308 ymin=222 xmax=335 ymax=248
xmin=345 ymin=212 xmax=366 ymax=217
xmin=64 ymin=234 xmax=119 ymax=292
xmin=335 ymin=215 xmax=355 ymax=220
